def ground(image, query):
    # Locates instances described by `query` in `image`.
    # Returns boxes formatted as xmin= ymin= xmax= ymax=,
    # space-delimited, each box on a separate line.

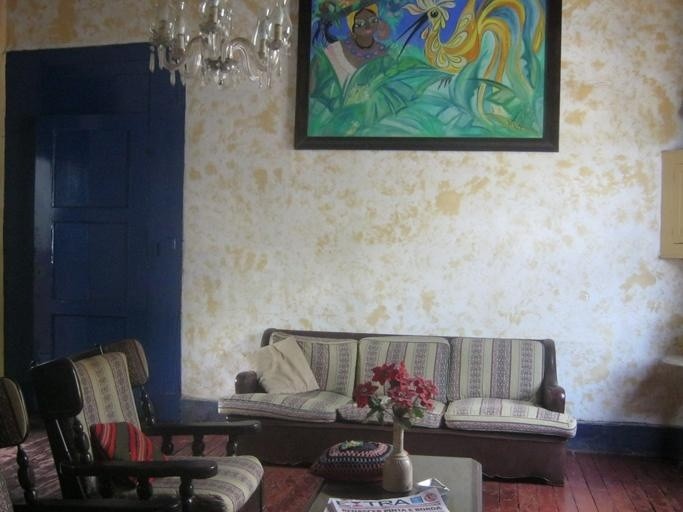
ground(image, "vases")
xmin=383 ymin=423 xmax=415 ymax=493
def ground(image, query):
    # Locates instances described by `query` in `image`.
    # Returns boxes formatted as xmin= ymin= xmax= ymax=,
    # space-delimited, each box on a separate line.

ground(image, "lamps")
xmin=146 ymin=1 xmax=293 ymax=92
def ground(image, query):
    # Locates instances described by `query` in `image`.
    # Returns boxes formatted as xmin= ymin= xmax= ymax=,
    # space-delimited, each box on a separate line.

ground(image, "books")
xmin=323 ymin=487 xmax=449 ymax=512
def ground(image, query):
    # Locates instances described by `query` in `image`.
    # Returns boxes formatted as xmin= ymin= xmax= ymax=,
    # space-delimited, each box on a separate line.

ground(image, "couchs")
xmin=216 ymin=327 xmax=577 ymax=487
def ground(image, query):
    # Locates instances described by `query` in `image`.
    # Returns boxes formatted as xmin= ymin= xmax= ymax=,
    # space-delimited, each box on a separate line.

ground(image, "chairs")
xmin=1 ymin=376 xmax=179 ymax=511
xmin=25 ymin=333 xmax=264 ymax=511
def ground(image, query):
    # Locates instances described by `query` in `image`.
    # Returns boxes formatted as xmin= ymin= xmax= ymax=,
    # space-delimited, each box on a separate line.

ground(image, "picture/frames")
xmin=293 ymin=1 xmax=564 ymax=153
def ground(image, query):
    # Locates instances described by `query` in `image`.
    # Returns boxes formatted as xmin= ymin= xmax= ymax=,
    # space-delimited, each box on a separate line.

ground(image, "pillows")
xmin=307 ymin=439 xmax=393 ymax=482
xmin=238 ymin=335 xmax=320 ymax=397
xmin=448 ymin=336 xmax=545 ymax=402
xmin=269 ymin=331 xmax=359 ymax=398
xmin=73 ymin=351 xmax=141 ymax=495
xmin=87 ymin=421 xmax=168 ymax=499
xmin=356 ymin=335 xmax=450 ymax=403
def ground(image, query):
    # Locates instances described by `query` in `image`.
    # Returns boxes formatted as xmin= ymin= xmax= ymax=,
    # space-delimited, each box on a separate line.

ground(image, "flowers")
xmin=352 ymin=361 xmax=439 ymax=431
